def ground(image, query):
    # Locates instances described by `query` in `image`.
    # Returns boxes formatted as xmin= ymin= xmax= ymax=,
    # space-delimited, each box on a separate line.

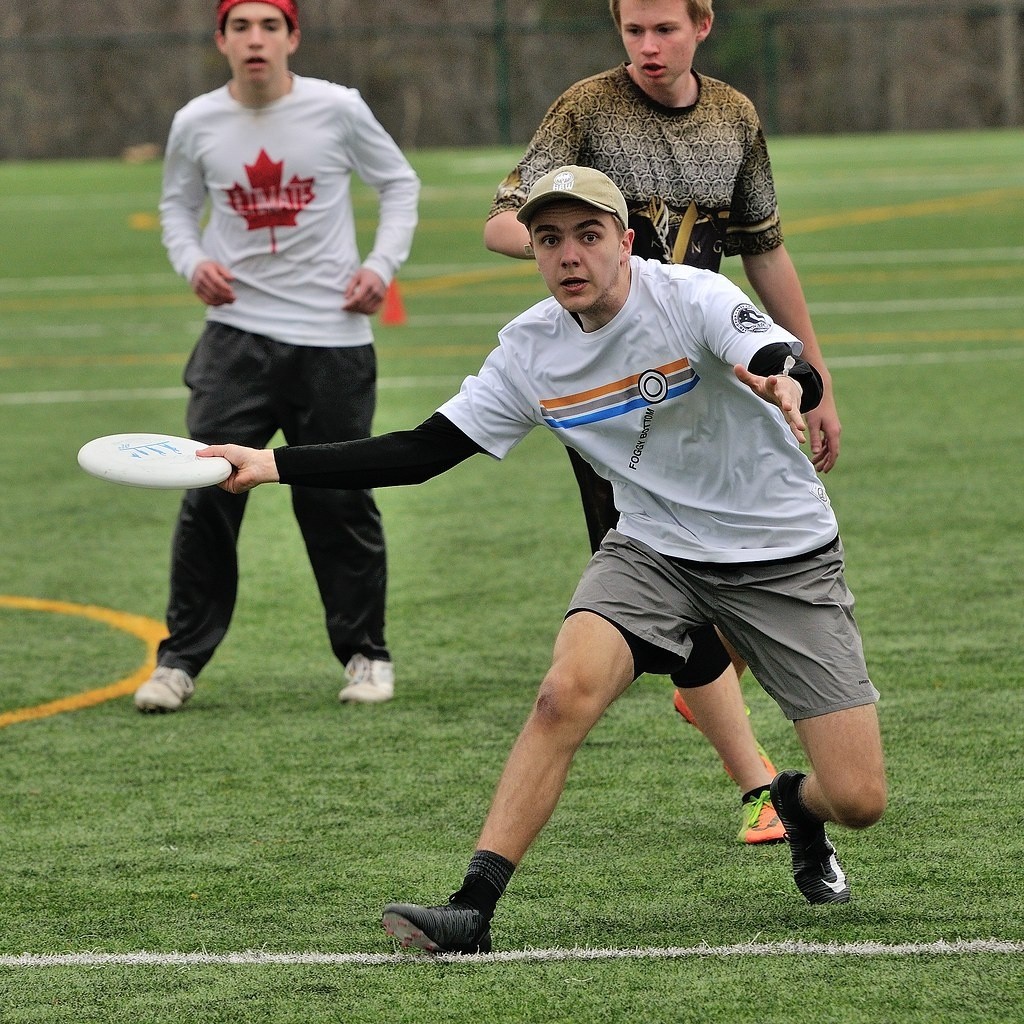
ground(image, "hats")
xmin=515 ymin=165 xmax=630 ymax=230
xmin=215 ymin=0 xmax=299 ymax=31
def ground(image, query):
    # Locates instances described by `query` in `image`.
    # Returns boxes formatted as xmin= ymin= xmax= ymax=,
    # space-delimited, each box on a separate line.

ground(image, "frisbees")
xmin=77 ymin=434 xmax=235 ymax=493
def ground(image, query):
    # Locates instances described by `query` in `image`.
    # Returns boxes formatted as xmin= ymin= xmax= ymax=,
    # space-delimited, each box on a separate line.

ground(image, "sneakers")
xmin=336 ymin=652 xmax=398 ymax=703
xmin=671 ymin=687 xmax=791 ymax=844
xmin=769 ymin=768 xmax=851 ymax=906
xmin=132 ymin=665 xmax=195 ymax=714
xmin=381 ymin=884 xmax=493 ymax=954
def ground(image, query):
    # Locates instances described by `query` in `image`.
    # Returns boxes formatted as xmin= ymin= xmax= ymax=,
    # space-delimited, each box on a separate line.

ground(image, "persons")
xmin=134 ymin=0 xmax=422 ymax=715
xmin=196 ymin=165 xmax=887 ymax=954
xmin=484 ymin=0 xmax=842 ymax=846
